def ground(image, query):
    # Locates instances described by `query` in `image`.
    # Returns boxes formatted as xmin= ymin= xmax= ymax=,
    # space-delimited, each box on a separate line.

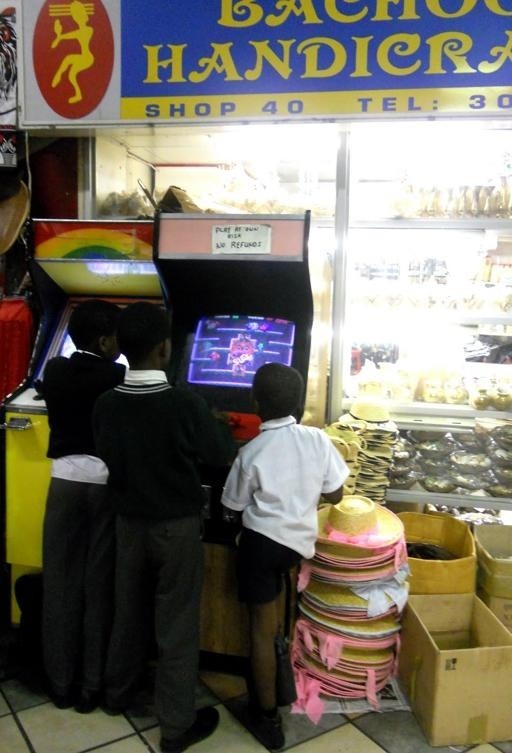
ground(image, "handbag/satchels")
xmin=319 ymin=419 xmax=367 ymax=495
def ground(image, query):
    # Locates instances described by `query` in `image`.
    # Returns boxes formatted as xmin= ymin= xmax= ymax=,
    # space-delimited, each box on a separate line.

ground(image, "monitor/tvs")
xmin=181 ymin=304 xmax=302 ymax=393
xmin=49 ymin=304 xmax=130 ymax=373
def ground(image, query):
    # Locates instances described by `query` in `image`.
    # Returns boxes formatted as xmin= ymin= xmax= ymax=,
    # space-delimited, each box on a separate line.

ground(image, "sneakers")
xmin=48 ymin=681 xmax=142 ymax=718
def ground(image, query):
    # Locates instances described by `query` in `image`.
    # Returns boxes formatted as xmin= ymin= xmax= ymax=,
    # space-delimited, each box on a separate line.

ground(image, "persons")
xmin=220 ymin=361 xmax=350 ymax=751
xmin=96 ymin=298 xmax=226 ymax=753
xmin=31 ymin=298 xmax=128 ymax=714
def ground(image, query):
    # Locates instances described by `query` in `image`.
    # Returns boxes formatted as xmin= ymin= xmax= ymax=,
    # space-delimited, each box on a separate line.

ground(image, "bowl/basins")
xmin=392 ymin=421 xmax=511 ymax=499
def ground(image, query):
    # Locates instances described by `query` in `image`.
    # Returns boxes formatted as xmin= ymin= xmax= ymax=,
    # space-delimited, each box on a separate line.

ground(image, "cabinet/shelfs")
xmin=87 ymin=116 xmax=512 ymax=511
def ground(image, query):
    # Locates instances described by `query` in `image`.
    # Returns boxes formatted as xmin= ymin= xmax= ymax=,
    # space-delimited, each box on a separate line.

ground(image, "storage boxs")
xmin=395 ymin=512 xmax=512 ymax=747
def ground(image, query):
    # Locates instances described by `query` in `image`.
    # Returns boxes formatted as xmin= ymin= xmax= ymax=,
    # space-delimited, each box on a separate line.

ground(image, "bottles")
xmin=415 ymin=373 xmax=509 ymax=411
xmin=418 ymin=173 xmax=511 ymax=217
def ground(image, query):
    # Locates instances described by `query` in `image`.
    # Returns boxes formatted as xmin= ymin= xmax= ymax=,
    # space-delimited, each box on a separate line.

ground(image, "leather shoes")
xmin=275 ymin=641 xmax=299 ymax=707
xmin=160 ymin=704 xmax=220 ymax=752
xmin=239 ymin=701 xmax=287 ymax=750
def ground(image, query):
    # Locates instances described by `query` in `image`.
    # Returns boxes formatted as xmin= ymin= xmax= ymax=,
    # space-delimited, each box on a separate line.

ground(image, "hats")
xmin=340 ymin=395 xmax=400 ymax=506
xmin=294 ymin=495 xmax=406 ymax=698
